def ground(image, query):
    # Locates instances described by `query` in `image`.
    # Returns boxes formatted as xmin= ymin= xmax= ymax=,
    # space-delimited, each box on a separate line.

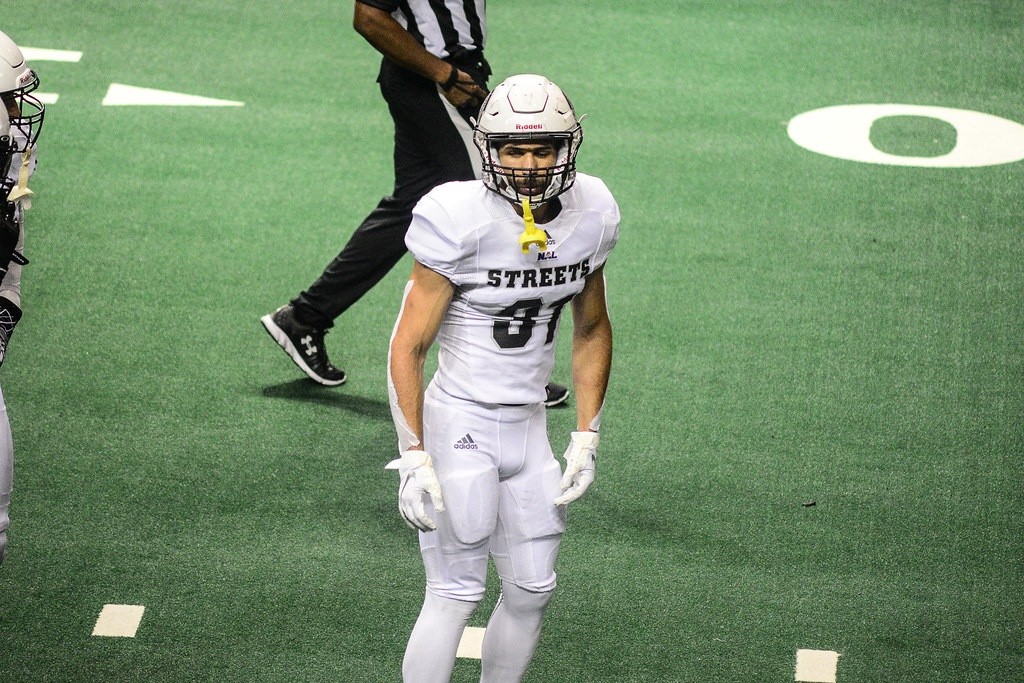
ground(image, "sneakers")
xmin=259 ymin=304 xmax=346 ymax=386
xmin=544 ymin=383 xmax=570 ymax=408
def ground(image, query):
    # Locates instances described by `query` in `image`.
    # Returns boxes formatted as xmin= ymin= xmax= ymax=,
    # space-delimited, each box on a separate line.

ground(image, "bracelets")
xmin=438 ymin=63 xmax=459 ymax=91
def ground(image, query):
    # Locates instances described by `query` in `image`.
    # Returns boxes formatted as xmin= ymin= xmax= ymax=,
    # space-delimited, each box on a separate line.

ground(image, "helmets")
xmin=474 ymin=74 xmax=583 ymax=210
xmin=0 ymin=30 xmax=45 ymax=153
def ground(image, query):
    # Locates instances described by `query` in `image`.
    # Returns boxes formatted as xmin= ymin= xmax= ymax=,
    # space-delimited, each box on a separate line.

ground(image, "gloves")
xmin=384 ymin=451 xmax=445 ymax=532
xmin=554 ymin=431 xmax=600 ymax=507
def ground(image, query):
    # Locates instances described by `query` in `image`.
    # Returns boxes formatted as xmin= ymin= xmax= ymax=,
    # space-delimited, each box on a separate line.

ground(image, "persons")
xmin=385 ymin=74 xmax=622 ymax=683
xmin=0 ymin=29 xmax=37 ymax=571
xmin=260 ymin=0 xmax=570 ymax=409
